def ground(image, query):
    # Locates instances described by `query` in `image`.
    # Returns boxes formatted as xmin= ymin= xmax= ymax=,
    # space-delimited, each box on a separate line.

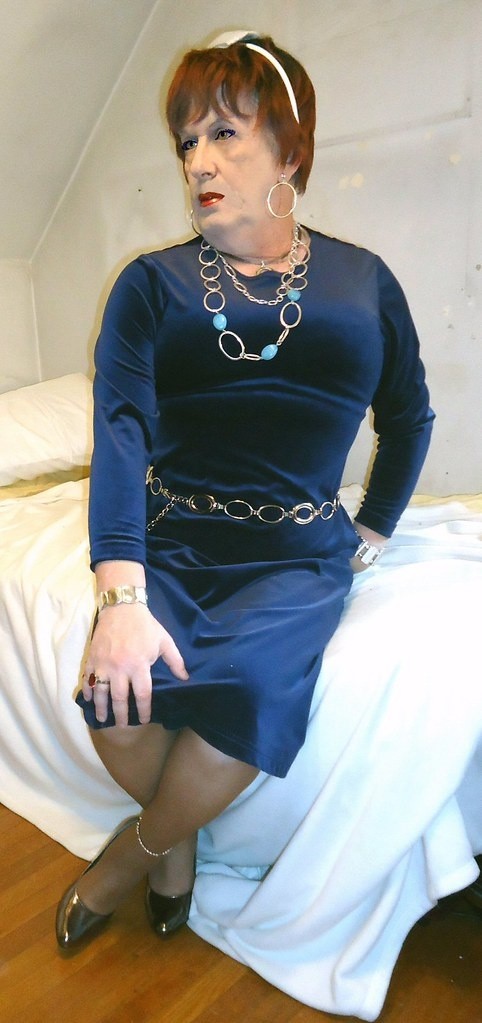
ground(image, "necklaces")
xmin=198 ymin=221 xmax=310 ymax=365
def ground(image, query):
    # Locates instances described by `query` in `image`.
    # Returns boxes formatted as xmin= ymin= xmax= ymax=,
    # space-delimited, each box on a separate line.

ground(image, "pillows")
xmin=0 ymin=373 xmax=96 ymax=497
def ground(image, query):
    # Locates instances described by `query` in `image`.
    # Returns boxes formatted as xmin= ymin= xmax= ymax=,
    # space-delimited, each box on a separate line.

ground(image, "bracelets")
xmin=353 ymin=530 xmax=384 ymax=566
xmin=97 ymin=581 xmax=148 ymax=610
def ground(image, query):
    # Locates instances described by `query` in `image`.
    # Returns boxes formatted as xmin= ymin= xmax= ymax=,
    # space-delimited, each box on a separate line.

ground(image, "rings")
xmin=90 ymin=672 xmax=109 ymax=686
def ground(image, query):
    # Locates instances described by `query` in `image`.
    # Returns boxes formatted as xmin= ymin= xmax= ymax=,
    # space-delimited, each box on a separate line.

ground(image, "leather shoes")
xmin=55 ymin=816 xmax=142 ymax=950
xmin=145 ymin=832 xmax=199 ymax=938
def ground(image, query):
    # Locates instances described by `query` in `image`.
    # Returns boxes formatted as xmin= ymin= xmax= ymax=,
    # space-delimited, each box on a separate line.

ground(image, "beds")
xmin=0 ymin=464 xmax=482 ymax=1023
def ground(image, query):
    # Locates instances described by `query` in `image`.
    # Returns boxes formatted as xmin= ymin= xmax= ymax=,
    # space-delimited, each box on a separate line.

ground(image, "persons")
xmin=12 ymin=32 xmax=437 ymax=945
